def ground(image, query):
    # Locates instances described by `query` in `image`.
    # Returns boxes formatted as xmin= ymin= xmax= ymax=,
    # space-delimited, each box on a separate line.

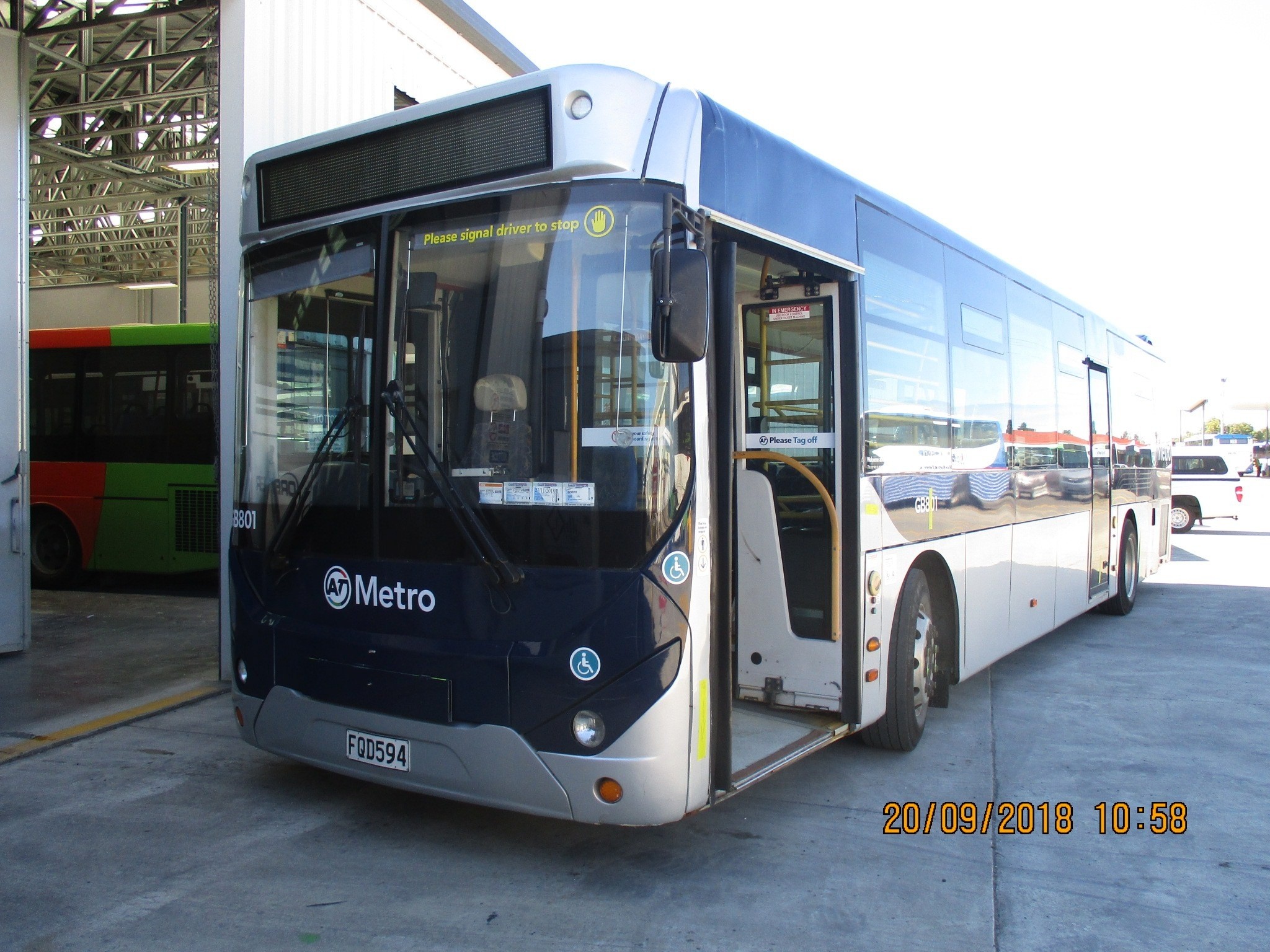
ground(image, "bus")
xmin=222 ymin=62 xmax=1177 ymax=832
xmin=1182 ymin=433 xmax=1254 ymax=479
xmin=25 ymin=320 xmax=221 ymax=586
xmin=864 ymin=409 xmax=1011 ymax=510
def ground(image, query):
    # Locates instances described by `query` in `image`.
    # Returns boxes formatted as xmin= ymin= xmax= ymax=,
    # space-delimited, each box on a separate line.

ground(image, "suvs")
xmin=1166 ymin=446 xmax=1245 ymax=534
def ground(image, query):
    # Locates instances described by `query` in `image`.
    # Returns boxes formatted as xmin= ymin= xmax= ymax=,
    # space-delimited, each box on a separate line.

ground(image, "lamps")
xmin=154 ymin=157 xmax=219 ymax=174
xmin=114 ymin=280 xmax=179 ymax=291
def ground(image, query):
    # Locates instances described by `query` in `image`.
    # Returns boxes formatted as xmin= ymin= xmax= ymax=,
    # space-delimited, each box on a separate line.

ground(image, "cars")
xmin=1059 ymin=468 xmax=1094 ymax=497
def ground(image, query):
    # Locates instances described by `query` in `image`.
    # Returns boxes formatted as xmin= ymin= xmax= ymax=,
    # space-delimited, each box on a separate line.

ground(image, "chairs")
xmin=467 ymin=372 xmax=534 ymax=485
xmin=121 ymin=402 xmax=147 ymax=436
xmin=189 ymin=402 xmax=213 ymax=431
xmin=149 ymin=406 xmax=167 ymax=436
